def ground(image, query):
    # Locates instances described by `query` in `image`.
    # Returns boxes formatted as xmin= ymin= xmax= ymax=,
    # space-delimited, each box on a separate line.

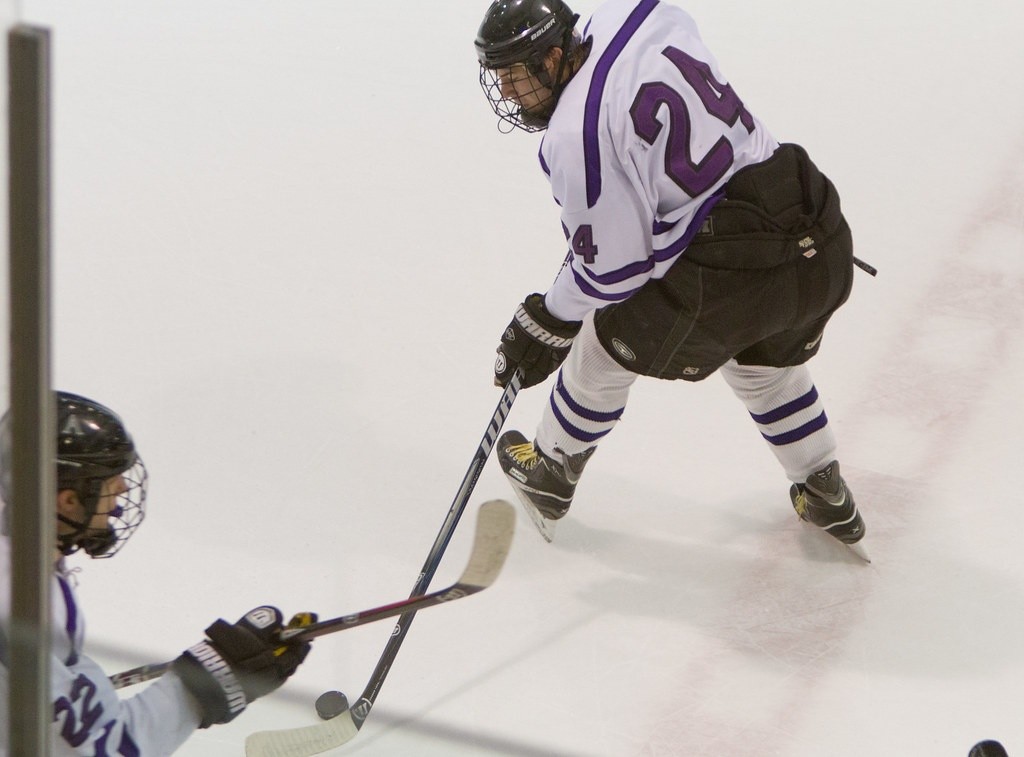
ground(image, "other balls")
xmin=315 ymin=689 xmax=349 ymax=720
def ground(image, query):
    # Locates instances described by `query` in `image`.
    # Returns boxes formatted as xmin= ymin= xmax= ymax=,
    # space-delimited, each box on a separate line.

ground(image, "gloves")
xmin=491 ymin=292 xmax=584 ymax=390
xmin=167 ymin=606 xmax=319 ymax=731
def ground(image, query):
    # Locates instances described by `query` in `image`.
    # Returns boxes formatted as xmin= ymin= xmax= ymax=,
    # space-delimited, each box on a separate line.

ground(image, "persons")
xmin=475 ymin=0 xmax=872 ymax=563
xmin=0 ymin=391 xmax=318 ymax=757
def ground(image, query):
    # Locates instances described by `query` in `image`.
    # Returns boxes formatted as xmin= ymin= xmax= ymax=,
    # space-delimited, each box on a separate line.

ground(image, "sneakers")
xmin=497 ymin=430 xmax=598 ymax=543
xmin=788 ymin=458 xmax=872 ymax=563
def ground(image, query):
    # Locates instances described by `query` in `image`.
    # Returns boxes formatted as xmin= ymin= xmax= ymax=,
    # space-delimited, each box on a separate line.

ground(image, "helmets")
xmin=475 ymin=0 xmax=595 ymax=134
xmin=0 ymin=388 xmax=151 ymax=561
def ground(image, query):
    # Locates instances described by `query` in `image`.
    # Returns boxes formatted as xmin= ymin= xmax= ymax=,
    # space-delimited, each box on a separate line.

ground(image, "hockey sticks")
xmin=244 ymin=250 xmax=572 ymax=757
xmin=95 ymin=488 xmax=518 ymax=699
